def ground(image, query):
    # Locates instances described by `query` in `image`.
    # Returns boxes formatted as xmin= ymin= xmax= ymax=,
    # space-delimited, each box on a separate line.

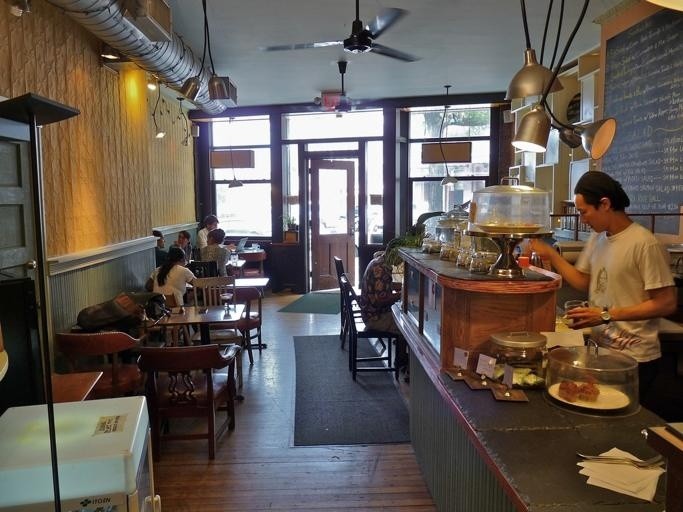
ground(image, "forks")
xmin=575 ymin=451 xmax=667 ymax=469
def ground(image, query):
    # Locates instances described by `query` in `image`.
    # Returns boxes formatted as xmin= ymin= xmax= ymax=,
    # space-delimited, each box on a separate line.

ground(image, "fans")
xmin=254 ymin=1 xmax=424 ymax=66
xmin=299 ymin=61 xmax=382 ymax=116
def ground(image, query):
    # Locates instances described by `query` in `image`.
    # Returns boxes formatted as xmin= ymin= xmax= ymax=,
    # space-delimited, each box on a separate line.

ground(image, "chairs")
xmin=140 ymin=343 xmax=241 ymax=460
xmin=339 ymin=271 xmax=401 ymax=383
xmin=125 ymin=242 xmax=268 ymax=365
xmin=53 ymin=330 xmax=147 ymax=403
xmin=331 ymin=252 xmax=361 ymax=348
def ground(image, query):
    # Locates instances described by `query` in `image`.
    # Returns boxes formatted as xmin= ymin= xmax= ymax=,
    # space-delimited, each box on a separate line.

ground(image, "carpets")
xmin=276 ymin=289 xmax=353 ymax=318
xmin=291 ymin=330 xmax=414 ymax=450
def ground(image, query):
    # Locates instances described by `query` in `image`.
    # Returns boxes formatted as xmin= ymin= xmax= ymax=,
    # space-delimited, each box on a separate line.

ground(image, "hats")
xmin=574 ymin=171 xmax=631 ymax=207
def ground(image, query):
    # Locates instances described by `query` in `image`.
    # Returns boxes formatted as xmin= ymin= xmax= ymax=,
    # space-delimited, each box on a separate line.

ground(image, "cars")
xmin=322 ymin=209 xmax=383 ymax=257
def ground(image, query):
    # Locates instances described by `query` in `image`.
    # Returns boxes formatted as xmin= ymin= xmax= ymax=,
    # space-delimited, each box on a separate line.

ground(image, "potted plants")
xmin=280 ymin=212 xmax=298 ymax=241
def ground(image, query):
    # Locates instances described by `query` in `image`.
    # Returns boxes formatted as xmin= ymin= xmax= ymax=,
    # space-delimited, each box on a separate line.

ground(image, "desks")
xmin=72 ymin=304 xmax=245 ymax=402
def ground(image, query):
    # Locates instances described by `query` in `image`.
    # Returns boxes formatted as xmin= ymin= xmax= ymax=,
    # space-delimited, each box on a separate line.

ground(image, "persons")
xmin=359 ymin=238 xmax=410 ymax=383
xmin=174 ymin=230 xmax=192 ymax=264
xmin=195 ymin=215 xmax=235 ymax=252
xmin=144 ymin=246 xmax=200 ymax=335
xmin=153 ymin=230 xmax=169 ymax=268
xmin=200 ymin=229 xmax=231 ymax=277
xmin=528 ymin=170 xmax=679 ymax=409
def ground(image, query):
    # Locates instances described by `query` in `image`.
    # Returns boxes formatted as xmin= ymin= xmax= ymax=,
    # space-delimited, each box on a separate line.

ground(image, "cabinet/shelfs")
xmin=509 ymin=44 xmax=603 ymax=227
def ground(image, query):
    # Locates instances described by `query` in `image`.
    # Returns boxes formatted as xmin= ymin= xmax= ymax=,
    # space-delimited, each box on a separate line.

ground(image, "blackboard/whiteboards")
xmin=595 ymin=0 xmax=683 ymax=215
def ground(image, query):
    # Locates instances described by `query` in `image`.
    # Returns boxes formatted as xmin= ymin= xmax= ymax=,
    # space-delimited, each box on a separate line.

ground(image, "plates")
xmin=548 ymin=380 xmax=633 ymax=410
xmin=478 ymin=224 xmax=544 ymax=234
xmin=567 ymin=94 xmax=581 ymax=123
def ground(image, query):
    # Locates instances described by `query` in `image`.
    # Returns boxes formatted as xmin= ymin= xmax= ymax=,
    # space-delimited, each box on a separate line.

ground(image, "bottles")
xmin=455 ymin=246 xmax=496 ymax=273
xmin=439 ymin=243 xmax=450 ymax=259
xmin=421 ymin=238 xmax=430 ymax=254
xmin=531 ymin=251 xmax=540 ymax=266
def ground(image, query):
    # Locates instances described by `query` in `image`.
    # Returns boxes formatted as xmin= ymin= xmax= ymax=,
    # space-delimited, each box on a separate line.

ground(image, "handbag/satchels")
xmin=77 ymin=291 xmax=169 ymax=330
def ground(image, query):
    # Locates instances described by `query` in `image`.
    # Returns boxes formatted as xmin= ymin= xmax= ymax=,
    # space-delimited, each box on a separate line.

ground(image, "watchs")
xmin=600 ymin=307 xmax=611 ymax=324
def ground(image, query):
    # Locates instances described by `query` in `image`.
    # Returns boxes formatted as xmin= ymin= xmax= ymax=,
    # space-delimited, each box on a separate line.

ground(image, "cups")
xmin=559 ymin=205 xmax=590 ymax=233
xmin=231 ymin=255 xmax=238 ymax=262
xmin=565 ymin=300 xmax=589 ymax=330
xmin=430 ymin=241 xmax=440 ymax=253
xmin=518 ymin=256 xmax=529 ymax=267
xmin=252 ymin=243 xmax=257 ymax=248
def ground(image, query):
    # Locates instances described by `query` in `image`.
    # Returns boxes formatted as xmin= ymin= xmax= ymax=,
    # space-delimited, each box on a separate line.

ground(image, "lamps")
xmin=224 ymin=115 xmax=244 ymax=189
xmin=501 ymin=1 xmax=619 ymax=162
xmin=434 ymin=84 xmax=462 ymax=187
xmin=147 ymin=73 xmax=168 ymax=140
xmin=179 ymin=1 xmax=236 ymax=100
xmin=171 ymin=95 xmax=192 ymax=147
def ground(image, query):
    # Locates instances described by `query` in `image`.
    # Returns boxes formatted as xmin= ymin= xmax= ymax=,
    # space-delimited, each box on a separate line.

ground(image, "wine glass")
xmin=219 ymin=292 xmax=233 ymax=318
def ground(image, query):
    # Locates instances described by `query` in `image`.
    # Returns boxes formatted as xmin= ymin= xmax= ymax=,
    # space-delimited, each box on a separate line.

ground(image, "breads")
xmin=558 ymin=381 xmax=600 ymax=403
